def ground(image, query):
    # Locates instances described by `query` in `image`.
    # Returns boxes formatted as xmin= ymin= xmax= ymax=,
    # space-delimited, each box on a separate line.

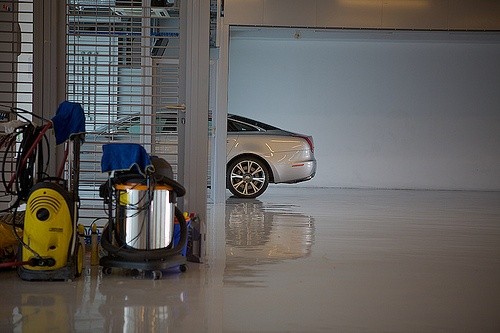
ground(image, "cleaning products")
xmin=78 ymin=224 xmax=86 ymax=269
xmin=90 ymin=222 xmax=99 ymax=268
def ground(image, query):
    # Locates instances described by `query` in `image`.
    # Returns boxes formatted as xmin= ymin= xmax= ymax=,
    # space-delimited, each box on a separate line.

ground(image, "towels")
xmin=100 ymin=142 xmax=155 ymax=176
xmin=50 ymin=100 xmax=86 ymax=145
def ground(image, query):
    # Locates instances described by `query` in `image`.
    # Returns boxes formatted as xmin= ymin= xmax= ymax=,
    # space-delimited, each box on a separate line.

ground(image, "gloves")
xmin=3 ymin=120 xmax=25 ymax=134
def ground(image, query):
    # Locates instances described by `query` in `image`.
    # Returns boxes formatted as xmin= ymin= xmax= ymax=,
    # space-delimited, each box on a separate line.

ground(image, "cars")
xmin=60 ymin=105 xmax=317 ymax=198
